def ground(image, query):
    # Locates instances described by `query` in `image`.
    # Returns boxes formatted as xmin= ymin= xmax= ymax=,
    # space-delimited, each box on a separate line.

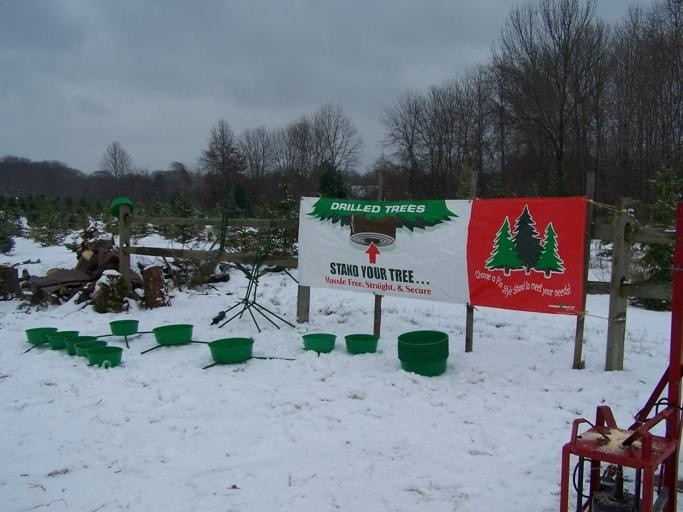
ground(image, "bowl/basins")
xmin=109 ymin=197 xmax=133 ymax=217
xmin=151 ymin=325 xmax=193 ymax=347
xmin=302 ymin=333 xmax=337 ymax=353
xmin=395 ymin=329 xmax=450 ymax=378
xmin=106 ymin=319 xmax=138 ymax=337
xmin=207 ymin=336 xmax=253 ymax=365
xmin=24 ymin=327 xmax=124 ymax=370
xmin=342 ymin=333 xmax=379 ymax=355
